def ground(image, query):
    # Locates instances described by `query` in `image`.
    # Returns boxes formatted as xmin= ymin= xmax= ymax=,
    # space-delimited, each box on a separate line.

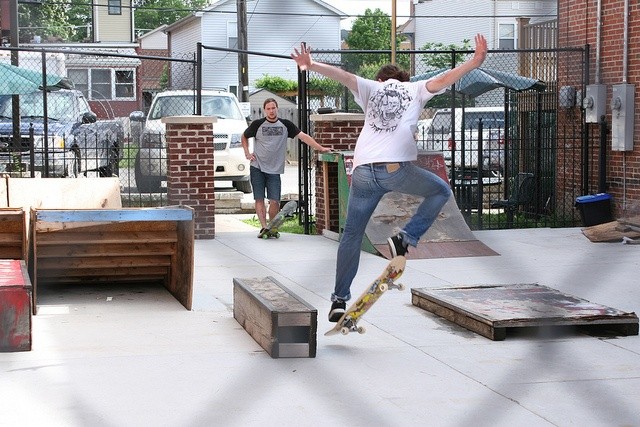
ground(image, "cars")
xmin=482 ymin=124 xmax=518 ymax=177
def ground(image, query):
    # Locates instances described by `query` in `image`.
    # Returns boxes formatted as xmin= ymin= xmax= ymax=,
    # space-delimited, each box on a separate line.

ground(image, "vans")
xmin=424 ymin=106 xmax=520 ymax=169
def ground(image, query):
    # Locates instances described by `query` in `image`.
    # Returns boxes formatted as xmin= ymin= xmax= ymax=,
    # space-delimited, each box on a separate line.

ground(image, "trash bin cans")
xmin=575 ymin=193 xmax=611 ymax=226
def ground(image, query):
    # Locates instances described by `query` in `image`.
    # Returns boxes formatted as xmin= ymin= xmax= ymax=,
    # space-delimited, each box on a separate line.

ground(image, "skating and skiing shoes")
xmin=328 ymin=300 xmax=345 ymax=322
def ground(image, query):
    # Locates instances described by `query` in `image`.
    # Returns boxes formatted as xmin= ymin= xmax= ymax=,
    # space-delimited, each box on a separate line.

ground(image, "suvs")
xmin=130 ymin=88 xmax=254 ymax=193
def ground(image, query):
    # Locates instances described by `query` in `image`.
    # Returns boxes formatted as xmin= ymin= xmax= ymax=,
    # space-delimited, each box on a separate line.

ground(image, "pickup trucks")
xmin=0 ymin=88 xmax=125 ymax=180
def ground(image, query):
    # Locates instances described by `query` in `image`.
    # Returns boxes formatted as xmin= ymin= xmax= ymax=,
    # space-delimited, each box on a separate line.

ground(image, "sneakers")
xmin=387 ymin=233 xmax=408 ymax=258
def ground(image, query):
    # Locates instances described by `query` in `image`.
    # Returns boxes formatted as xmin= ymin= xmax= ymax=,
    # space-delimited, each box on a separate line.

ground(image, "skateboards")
xmin=256 ymin=200 xmax=297 ymax=238
xmin=325 ymin=255 xmax=406 ymax=336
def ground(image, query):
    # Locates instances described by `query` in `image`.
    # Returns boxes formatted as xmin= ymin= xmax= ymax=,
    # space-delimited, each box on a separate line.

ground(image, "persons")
xmin=291 ymin=33 xmax=487 ymax=323
xmin=242 ymin=98 xmax=333 ymax=238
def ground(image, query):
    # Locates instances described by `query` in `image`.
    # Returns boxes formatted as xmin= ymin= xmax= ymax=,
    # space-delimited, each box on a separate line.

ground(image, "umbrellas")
xmin=0 ymin=61 xmax=75 ymax=97
xmin=403 ymin=62 xmax=548 ymax=213
